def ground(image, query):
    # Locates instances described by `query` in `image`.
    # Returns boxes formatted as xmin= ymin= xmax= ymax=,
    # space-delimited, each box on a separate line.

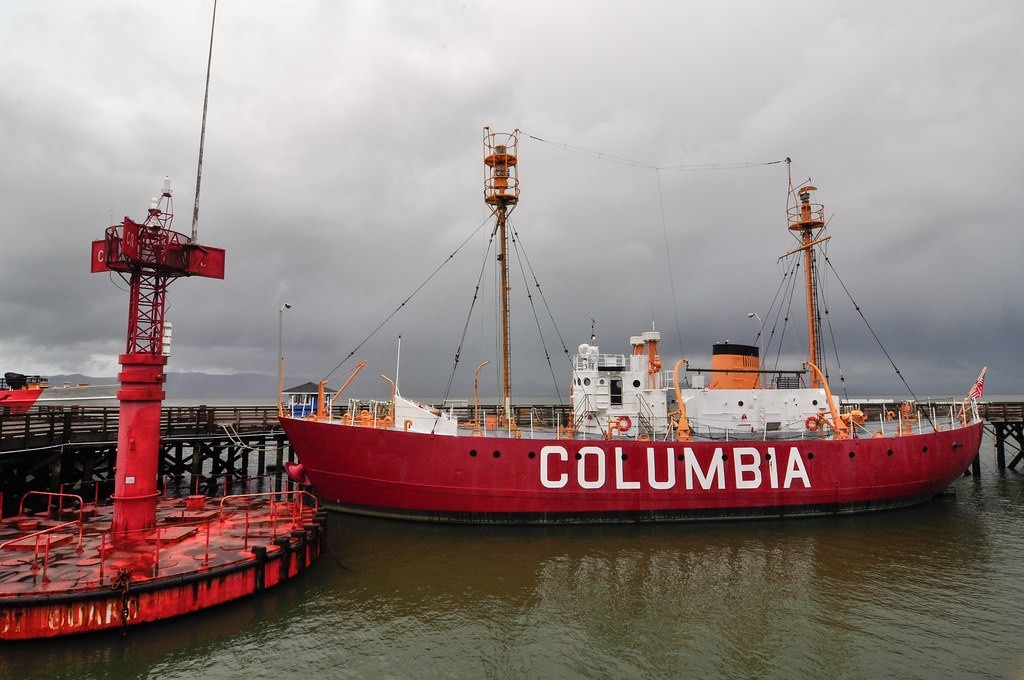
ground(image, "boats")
xmin=278 ymin=127 xmax=988 ymax=526
xmin=0 ymin=372 xmax=49 ymax=414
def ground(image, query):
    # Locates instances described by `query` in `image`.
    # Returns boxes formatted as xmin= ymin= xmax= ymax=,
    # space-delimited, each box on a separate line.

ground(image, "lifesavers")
xmin=617 ymin=415 xmax=632 ymax=431
xmin=805 ymin=416 xmax=820 ymax=432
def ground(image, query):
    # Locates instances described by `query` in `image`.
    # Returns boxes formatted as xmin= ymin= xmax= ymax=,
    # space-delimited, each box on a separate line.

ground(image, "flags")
xmin=968 ymin=368 xmax=986 ymax=399
